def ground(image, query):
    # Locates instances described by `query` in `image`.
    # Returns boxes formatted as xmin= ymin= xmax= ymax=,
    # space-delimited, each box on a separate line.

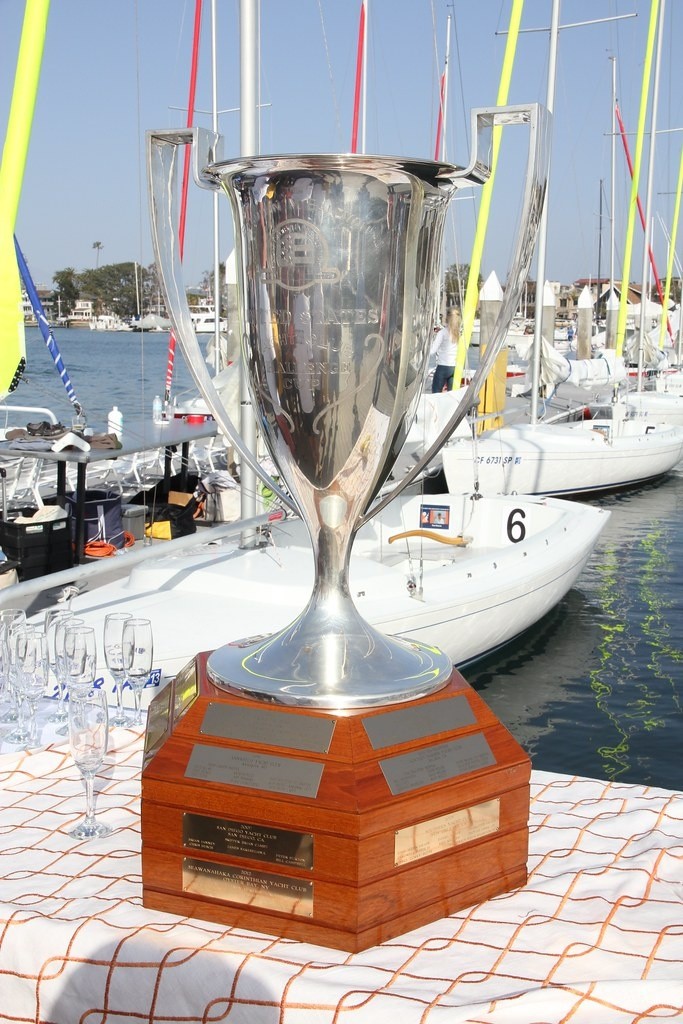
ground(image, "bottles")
xmin=108 ymin=406 xmax=123 ymax=441
xmin=153 ymin=396 xmax=162 ymax=422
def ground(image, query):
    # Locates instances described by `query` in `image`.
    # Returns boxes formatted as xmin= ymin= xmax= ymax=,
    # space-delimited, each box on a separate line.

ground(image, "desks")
xmin=0 ymin=418 xmax=229 ymax=513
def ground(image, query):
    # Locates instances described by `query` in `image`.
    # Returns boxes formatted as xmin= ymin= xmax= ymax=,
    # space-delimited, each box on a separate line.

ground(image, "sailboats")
xmin=21 ymin=0 xmax=613 ymax=724
xmin=413 ymin=0 xmax=681 ymax=499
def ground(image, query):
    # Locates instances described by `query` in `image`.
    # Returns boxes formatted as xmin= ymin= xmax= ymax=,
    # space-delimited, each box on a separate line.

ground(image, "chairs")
xmin=1 ymin=396 xmax=215 ymax=521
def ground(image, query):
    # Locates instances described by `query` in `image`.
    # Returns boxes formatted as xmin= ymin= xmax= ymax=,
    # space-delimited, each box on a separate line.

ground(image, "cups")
xmin=165 ymin=405 xmax=174 ymax=420
xmin=72 ymin=416 xmax=84 ymax=431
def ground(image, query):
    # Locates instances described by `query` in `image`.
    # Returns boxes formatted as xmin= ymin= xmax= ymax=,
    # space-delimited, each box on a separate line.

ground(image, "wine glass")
xmin=4 ymin=624 xmax=39 ymax=743
xmin=0 ymin=609 xmax=26 ymax=724
xmin=64 ymin=627 xmax=96 ymax=731
xmin=104 ymin=612 xmax=135 ymax=727
xmin=44 ymin=610 xmax=74 ymax=723
xmin=122 ymin=619 xmax=153 ymax=727
xmin=0 ymin=623 xmax=26 ymax=702
xmin=16 ymin=633 xmax=48 ymax=751
xmin=68 ymin=689 xmax=112 ymax=840
xmin=55 ymin=619 xmax=89 ymax=736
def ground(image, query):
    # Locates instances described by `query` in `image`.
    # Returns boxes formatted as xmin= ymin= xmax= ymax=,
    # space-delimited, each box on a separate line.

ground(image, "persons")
xmin=431 ymin=308 xmax=461 ymax=392
xmin=434 ymin=513 xmax=444 ymax=524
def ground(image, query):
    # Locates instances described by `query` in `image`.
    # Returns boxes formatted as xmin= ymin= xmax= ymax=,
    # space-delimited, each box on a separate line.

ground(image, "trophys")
xmin=141 ymin=102 xmax=552 ymax=951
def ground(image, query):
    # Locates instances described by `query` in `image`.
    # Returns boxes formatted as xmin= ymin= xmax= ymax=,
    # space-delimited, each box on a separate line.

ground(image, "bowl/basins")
xmin=187 ymin=414 xmax=204 ymax=423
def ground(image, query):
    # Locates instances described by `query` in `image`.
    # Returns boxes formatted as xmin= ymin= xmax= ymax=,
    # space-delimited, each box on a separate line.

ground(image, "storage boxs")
xmin=0 ymin=470 xmax=243 ymax=583
